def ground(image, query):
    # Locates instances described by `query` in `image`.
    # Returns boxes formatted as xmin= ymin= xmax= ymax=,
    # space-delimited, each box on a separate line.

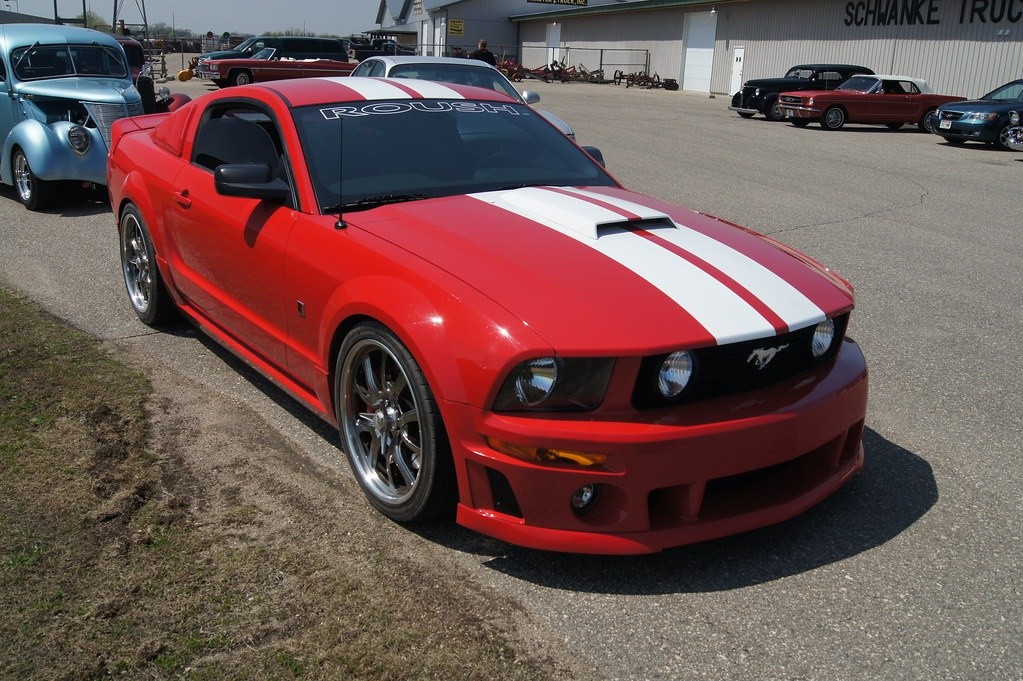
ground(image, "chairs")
xmin=394 ymin=112 xmax=469 ymax=176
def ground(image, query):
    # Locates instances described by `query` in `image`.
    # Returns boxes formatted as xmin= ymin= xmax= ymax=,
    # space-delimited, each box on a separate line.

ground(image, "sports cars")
xmin=103 ymin=71 xmax=873 ymax=556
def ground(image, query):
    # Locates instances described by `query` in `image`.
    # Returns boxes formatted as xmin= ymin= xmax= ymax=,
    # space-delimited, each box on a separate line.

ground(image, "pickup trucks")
xmin=338 ymin=36 xmax=417 ymax=64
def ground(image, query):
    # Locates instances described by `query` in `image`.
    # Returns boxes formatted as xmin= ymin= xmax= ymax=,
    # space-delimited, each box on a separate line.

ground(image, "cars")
xmin=327 ymin=55 xmax=576 ymax=160
xmin=0 ymin=23 xmax=148 ymax=211
xmin=67 ymin=30 xmax=171 ymax=114
xmin=929 ymin=78 xmax=1023 ymax=153
xmin=196 ymin=34 xmax=349 ymax=77
xmin=196 ymin=47 xmax=364 ymax=92
xmin=774 ymin=73 xmax=968 ymax=133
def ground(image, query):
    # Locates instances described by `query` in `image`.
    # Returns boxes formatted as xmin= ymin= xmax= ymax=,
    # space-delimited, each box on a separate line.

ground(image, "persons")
xmin=346 ymin=43 xmax=357 ymax=59
xmin=468 ymin=39 xmax=497 ymax=68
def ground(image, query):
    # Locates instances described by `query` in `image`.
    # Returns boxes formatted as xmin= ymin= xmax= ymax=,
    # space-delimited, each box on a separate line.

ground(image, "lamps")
xmin=710 ymin=5 xmax=726 ymax=18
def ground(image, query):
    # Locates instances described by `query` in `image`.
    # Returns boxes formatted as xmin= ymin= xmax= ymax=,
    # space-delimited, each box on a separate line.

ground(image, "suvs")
xmin=727 ymin=64 xmax=879 ymax=122
